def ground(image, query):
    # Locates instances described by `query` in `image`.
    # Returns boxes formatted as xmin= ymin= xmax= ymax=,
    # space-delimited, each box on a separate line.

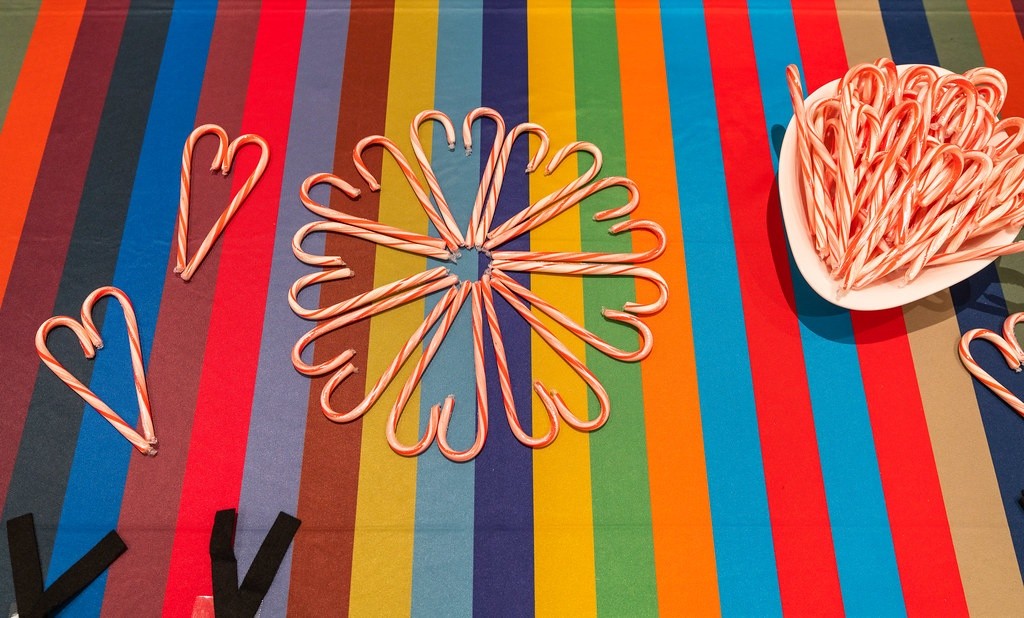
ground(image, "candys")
xmin=173 ymin=123 xmax=272 ymax=280
xmin=36 ymin=286 xmax=163 ymax=457
xmin=286 ymin=107 xmax=670 ymax=463
xmin=786 ymin=57 xmax=1023 ymax=416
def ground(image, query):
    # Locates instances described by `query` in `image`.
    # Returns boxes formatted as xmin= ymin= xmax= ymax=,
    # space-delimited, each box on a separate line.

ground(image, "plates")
xmin=775 ymin=64 xmax=1024 ymax=311
